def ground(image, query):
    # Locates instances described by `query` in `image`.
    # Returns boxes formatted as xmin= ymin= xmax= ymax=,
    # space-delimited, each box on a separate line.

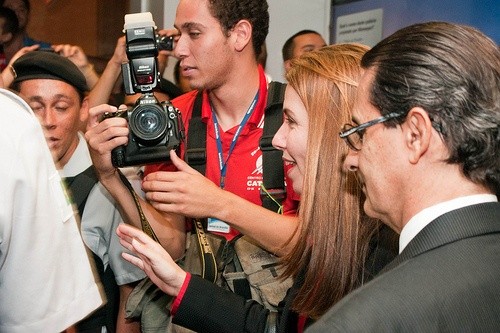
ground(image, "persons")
xmin=0 ymin=0 xmax=399 ymax=332
xmin=300 ymin=20 xmax=500 ymax=333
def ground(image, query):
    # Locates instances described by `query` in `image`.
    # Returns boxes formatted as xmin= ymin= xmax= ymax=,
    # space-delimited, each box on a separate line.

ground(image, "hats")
xmin=13 ymin=50 xmax=87 ymax=90
xmin=119 ymin=77 xmax=184 ymax=99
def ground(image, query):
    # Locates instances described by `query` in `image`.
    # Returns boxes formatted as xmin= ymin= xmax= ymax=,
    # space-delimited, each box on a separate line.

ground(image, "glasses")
xmin=339 ymin=111 xmax=406 ymax=151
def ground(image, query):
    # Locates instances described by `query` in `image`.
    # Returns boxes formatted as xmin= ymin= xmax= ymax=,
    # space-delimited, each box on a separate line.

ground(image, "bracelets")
xmin=82 ymin=64 xmax=95 ymax=77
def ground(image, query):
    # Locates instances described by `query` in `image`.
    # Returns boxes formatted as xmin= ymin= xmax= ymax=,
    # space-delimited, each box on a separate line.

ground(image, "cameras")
xmin=98 ymin=12 xmax=185 ymax=168
